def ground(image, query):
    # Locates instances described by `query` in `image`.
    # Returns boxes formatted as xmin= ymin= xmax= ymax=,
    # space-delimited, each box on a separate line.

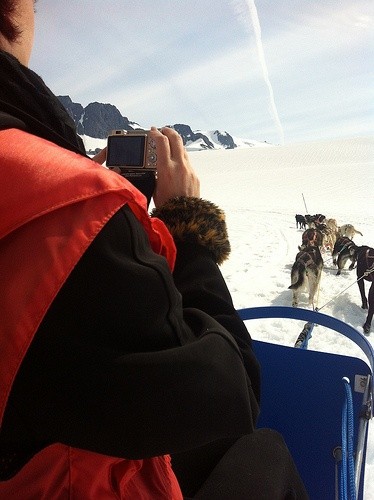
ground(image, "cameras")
xmin=106 ymin=130 xmax=162 ymax=177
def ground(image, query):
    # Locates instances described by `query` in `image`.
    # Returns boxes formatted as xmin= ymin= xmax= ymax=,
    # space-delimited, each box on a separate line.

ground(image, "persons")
xmin=0 ymin=0 xmax=313 ymax=499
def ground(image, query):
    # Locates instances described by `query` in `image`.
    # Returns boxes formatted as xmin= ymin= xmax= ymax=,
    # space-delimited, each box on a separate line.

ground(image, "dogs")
xmin=287 ymin=214 xmax=374 ymax=337
